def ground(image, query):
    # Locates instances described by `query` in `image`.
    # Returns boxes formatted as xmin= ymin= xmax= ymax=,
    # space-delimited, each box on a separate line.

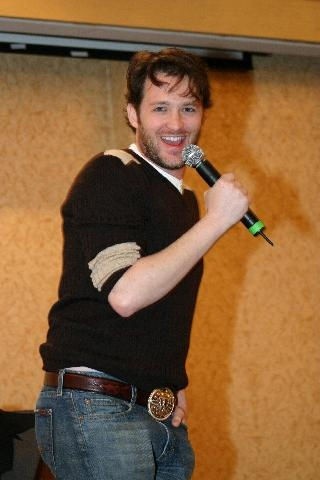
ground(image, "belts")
xmin=43 ymin=372 xmax=178 ymax=420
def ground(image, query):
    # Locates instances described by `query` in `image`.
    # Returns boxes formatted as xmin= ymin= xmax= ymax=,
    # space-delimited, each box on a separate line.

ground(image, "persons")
xmin=34 ymin=48 xmax=249 ymax=475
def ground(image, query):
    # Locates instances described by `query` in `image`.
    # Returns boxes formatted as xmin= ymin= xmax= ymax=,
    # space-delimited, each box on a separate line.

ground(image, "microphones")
xmin=182 ymin=144 xmax=274 ymax=248
